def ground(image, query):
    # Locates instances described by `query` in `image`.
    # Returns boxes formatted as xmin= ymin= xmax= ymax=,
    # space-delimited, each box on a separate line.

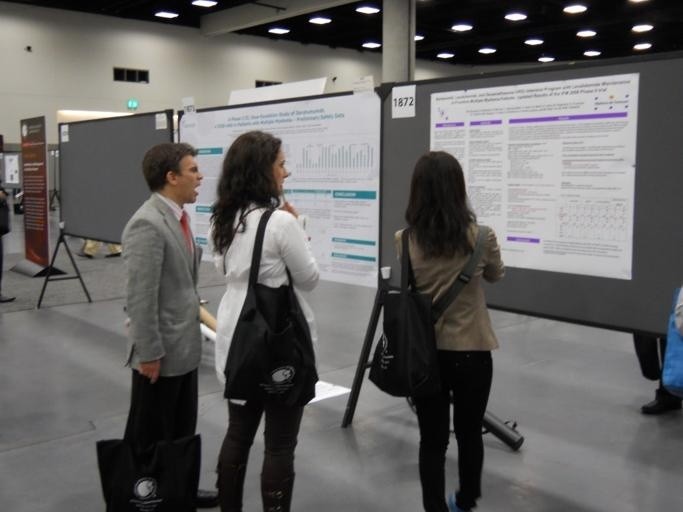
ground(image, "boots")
xmin=216 ymin=463 xmax=247 ymax=511
xmin=260 ymin=472 xmax=295 ymax=511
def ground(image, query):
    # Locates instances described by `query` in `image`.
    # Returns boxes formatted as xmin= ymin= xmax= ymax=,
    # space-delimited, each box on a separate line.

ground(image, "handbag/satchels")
xmin=93 ymin=433 xmax=203 ymax=511
xmin=367 ymin=292 xmax=439 ymax=397
xmin=219 ymin=282 xmax=319 ymax=408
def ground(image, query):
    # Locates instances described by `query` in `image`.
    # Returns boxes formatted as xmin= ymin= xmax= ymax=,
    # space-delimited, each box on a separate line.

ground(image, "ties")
xmin=179 ymin=213 xmax=192 ymax=256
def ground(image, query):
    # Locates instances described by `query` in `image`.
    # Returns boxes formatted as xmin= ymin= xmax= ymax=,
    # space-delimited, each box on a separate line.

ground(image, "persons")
xmin=393 ymin=151 xmax=505 ymax=512
xmin=205 ymin=130 xmax=318 ymax=512
xmin=640 ymin=278 xmax=682 ymax=414
xmin=0 ymin=134 xmax=16 ymax=302
xmin=75 ymin=238 xmax=121 ymax=259
xmin=121 ymin=141 xmax=219 ymax=509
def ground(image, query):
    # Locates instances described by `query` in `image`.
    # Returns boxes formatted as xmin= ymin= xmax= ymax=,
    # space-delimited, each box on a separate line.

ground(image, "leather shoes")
xmin=641 ymin=388 xmax=681 ymax=415
